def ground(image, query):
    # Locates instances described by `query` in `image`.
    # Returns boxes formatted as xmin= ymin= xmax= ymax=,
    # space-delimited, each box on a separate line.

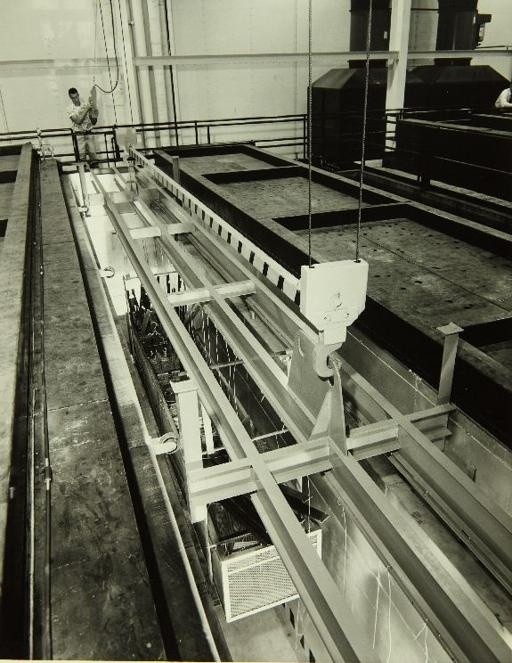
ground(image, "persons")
xmin=67 ymin=86 xmax=99 ymax=168
xmin=494 ymin=81 xmax=511 ymax=107
